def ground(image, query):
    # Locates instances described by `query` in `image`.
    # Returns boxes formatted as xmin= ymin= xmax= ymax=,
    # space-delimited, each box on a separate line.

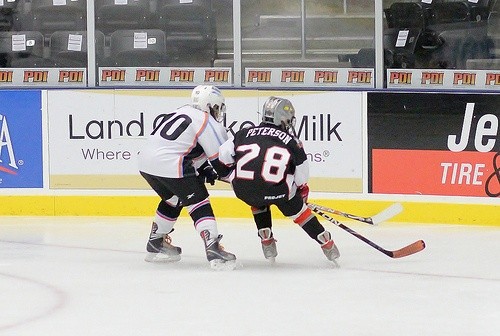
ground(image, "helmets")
xmin=261 ymin=97 xmax=295 ymax=126
xmin=191 ymin=84 xmax=225 ymax=114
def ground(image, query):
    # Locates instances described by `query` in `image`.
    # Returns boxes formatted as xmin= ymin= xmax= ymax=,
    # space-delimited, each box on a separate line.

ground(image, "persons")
xmin=137 ymin=84 xmax=236 ymax=269
xmin=219 ymin=95 xmax=342 ymax=270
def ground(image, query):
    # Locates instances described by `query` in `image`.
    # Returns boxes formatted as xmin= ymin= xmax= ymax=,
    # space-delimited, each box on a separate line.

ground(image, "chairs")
xmin=0 ymin=0 xmax=218 ymax=68
xmin=339 ymin=0 xmax=500 ymax=70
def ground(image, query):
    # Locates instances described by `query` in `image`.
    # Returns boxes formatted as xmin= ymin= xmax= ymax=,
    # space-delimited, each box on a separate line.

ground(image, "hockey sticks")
xmin=215 ymin=175 xmax=403 ymax=226
xmin=306 ymin=203 xmax=426 ymax=259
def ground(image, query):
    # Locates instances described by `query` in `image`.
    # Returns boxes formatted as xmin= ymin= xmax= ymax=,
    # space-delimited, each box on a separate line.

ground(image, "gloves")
xmin=298 ymin=184 xmax=309 ymax=202
xmin=202 ymin=167 xmax=217 ymax=185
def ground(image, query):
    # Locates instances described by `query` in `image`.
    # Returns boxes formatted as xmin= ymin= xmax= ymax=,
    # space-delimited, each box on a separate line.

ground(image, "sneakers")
xmin=259 ymin=228 xmax=277 ymax=263
xmin=317 ymin=231 xmax=340 ymax=267
xmin=200 ymin=230 xmax=236 ymax=271
xmin=145 ymin=222 xmax=181 ymax=261
